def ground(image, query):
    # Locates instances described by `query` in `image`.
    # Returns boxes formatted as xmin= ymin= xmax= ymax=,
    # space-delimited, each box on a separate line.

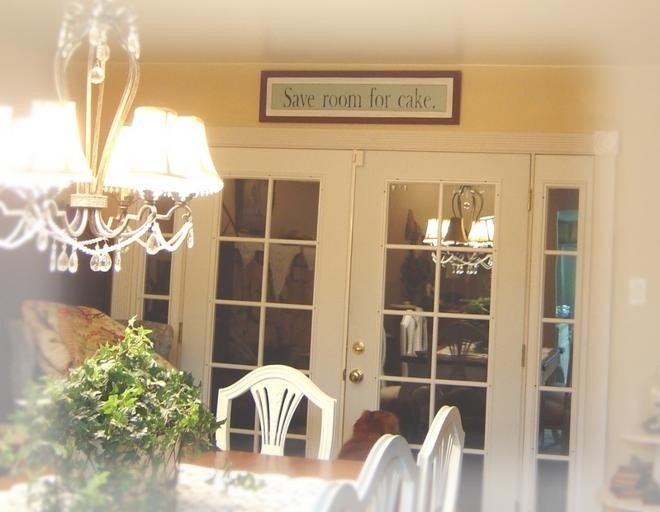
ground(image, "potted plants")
xmin=456 ymin=296 xmax=490 ymax=350
xmin=0 ymin=313 xmax=264 ymax=511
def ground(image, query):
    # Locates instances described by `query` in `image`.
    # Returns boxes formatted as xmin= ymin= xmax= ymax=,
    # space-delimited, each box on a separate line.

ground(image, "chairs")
xmin=541 ymin=341 xmax=574 ymax=452
xmin=420 ymin=405 xmax=468 ymax=511
xmin=379 ymin=306 xmax=427 ymax=443
xmin=213 ymin=363 xmax=335 ymax=463
xmin=328 ymin=484 xmax=364 ymax=512
xmin=361 ymin=431 xmax=423 ymax=512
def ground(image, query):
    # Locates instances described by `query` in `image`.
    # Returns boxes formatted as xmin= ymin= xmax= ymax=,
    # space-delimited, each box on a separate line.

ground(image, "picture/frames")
xmin=259 ymin=70 xmax=462 ymax=125
xmin=235 ymin=179 xmax=275 ymax=236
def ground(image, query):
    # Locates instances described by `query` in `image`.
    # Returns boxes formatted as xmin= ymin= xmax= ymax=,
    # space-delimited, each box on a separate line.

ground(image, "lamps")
xmin=0 ymin=1 xmax=227 ymax=277
xmin=423 ymin=183 xmax=496 ymax=275
